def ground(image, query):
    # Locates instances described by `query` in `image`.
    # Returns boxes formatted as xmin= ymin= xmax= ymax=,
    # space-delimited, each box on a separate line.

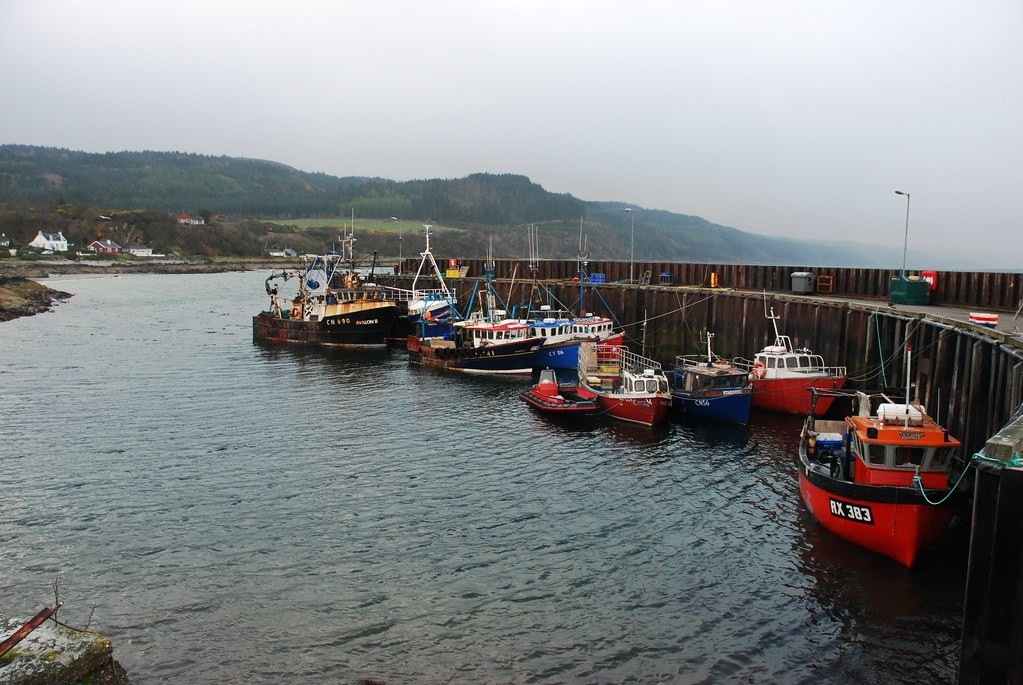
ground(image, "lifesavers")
xmin=292 ymin=308 xmax=298 ymax=317
xmin=434 ymin=346 xmax=486 ymax=359
xmin=753 ymin=361 xmax=767 ymax=378
xmin=715 ymin=360 xmax=732 ymax=365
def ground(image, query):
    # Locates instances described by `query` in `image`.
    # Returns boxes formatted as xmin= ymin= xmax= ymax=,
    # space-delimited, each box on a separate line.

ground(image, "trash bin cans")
xmin=791 ymin=272 xmax=816 ymax=294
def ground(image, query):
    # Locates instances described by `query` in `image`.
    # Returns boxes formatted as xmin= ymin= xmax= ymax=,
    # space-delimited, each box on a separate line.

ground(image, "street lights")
xmin=894 ymin=190 xmax=911 ymax=276
xmin=625 ymin=208 xmax=634 ymax=284
xmin=391 ymin=216 xmax=402 ymax=276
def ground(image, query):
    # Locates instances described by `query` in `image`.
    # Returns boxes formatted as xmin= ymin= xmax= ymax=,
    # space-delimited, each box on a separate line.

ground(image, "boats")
xmin=518 ymin=366 xmax=602 ymax=421
xmin=577 ymin=342 xmax=672 ymax=428
xmin=795 ymin=344 xmax=972 ymax=567
xmin=733 ymin=287 xmax=848 ymax=419
xmin=252 ymin=207 xmax=628 ymax=377
xmin=663 ymin=329 xmax=755 ymax=427
